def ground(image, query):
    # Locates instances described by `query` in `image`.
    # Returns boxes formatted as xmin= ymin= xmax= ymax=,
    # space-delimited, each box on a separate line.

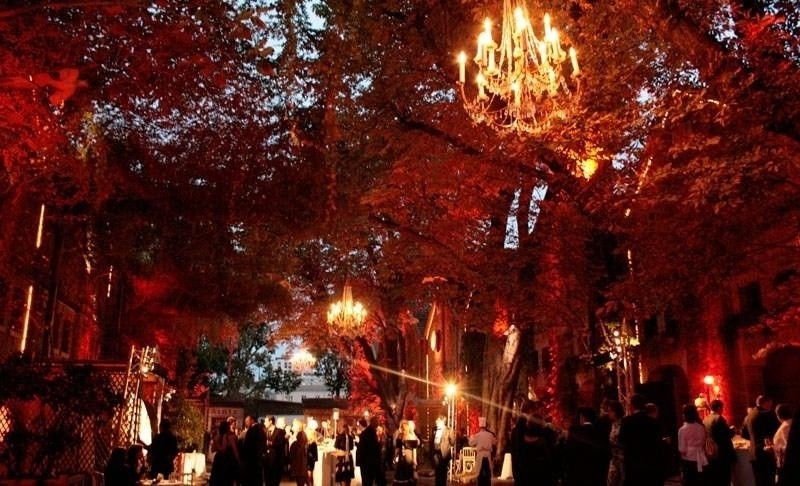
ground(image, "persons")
xmin=97 ymin=393 xmax=800 ymax=486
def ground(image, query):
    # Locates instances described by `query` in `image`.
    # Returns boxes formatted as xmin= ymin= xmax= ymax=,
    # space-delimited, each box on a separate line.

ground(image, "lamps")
xmin=326 ymin=278 xmax=367 ymax=340
xmin=452 ymin=0 xmax=582 ymax=136
